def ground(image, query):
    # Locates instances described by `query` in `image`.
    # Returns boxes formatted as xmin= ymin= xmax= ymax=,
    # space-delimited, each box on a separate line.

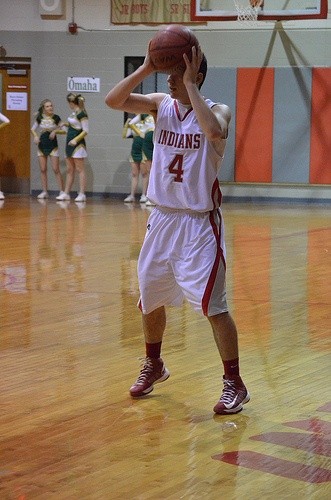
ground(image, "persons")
xmin=0 ymin=113 xmax=10 ymax=199
xmin=56 ymin=93 xmax=89 ymax=202
xmin=124 ymin=108 xmax=156 ymax=207
xmin=105 ymin=40 xmax=250 ymax=415
xmin=30 ymin=100 xmax=66 ymax=199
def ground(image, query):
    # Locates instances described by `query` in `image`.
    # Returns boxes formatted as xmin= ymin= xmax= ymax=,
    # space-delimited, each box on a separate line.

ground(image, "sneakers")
xmin=213 ymin=375 xmax=251 ymax=415
xmin=129 ymin=357 xmax=170 ymax=398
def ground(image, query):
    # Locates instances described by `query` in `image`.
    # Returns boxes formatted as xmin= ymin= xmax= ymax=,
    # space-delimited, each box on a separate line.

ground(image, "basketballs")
xmin=150 ymin=25 xmax=200 ymax=74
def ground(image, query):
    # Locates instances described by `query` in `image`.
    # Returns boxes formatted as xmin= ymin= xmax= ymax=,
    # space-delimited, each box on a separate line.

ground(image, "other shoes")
xmin=145 ymin=200 xmax=156 ymax=205
xmin=139 ymin=194 xmax=147 ymax=202
xmin=74 ymin=193 xmax=86 ymax=202
xmin=56 ymin=194 xmax=71 ymax=201
xmin=60 ymin=191 xmax=63 ymax=196
xmin=124 ymin=195 xmax=136 ymax=202
xmin=37 ymin=191 xmax=48 ymax=199
xmin=0 ymin=190 xmax=5 ymax=199
